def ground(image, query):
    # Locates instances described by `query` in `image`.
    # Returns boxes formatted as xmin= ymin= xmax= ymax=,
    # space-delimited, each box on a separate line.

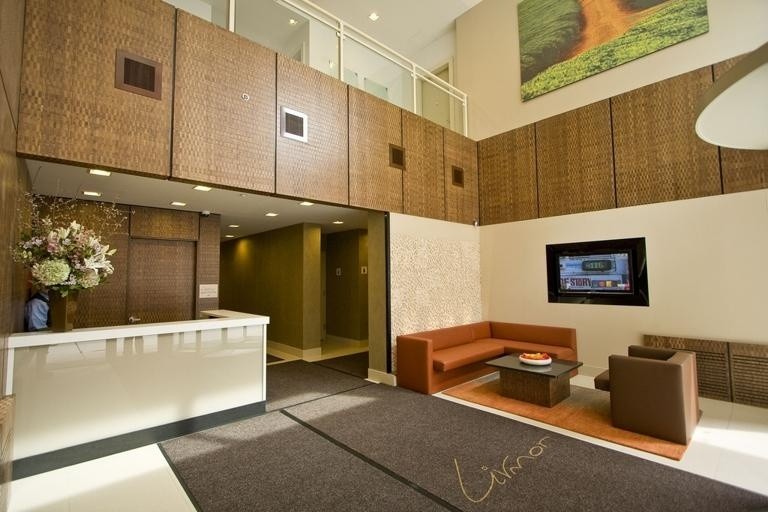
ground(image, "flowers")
xmin=13 ymin=166 xmax=136 ymax=298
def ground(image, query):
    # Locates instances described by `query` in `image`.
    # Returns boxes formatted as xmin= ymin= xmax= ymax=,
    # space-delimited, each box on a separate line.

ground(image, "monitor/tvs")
xmin=554 ymin=246 xmax=638 ymax=298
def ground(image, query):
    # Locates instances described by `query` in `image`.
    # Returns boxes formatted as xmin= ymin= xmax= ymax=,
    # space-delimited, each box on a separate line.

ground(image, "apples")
xmin=522 ymin=352 xmax=550 ymax=360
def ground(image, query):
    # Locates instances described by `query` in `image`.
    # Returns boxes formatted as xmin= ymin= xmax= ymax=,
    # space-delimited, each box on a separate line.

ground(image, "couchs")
xmin=397 ymin=320 xmax=577 ymax=396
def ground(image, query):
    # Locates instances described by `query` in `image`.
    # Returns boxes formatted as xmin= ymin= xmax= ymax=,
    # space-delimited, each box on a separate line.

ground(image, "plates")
xmin=518 ymin=355 xmax=552 ymax=366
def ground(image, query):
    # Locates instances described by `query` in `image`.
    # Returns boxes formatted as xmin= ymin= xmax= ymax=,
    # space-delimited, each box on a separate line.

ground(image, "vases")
xmin=47 ymin=287 xmax=80 ymax=333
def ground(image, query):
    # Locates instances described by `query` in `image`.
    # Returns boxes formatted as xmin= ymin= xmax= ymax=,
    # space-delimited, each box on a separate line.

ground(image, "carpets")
xmin=442 ymin=369 xmax=703 ymax=461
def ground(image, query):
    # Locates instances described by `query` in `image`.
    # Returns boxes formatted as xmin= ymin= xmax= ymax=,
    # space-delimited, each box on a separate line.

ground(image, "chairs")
xmin=595 ymin=343 xmax=699 ymax=445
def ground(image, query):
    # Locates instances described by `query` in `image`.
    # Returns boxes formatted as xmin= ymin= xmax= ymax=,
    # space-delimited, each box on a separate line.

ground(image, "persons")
xmin=24 ymin=286 xmax=50 ymax=332
